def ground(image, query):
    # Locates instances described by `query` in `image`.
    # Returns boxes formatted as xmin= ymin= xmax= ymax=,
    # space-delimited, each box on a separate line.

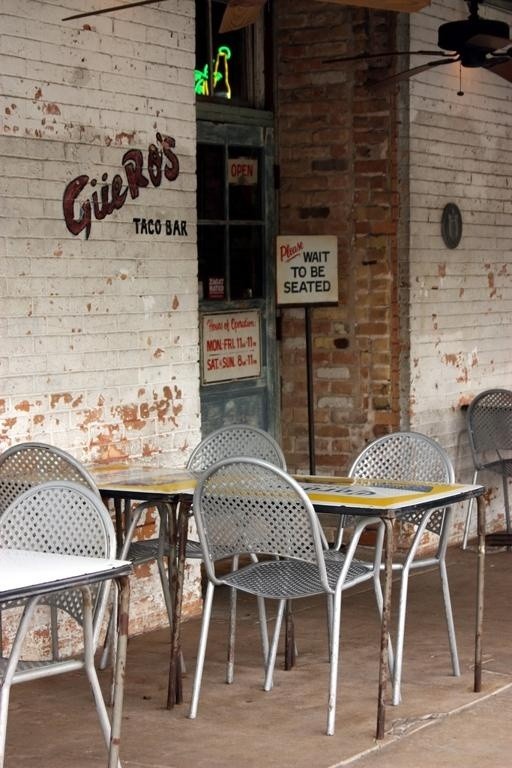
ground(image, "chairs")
xmin=1 ymin=480 xmax=124 ymax=768
xmin=462 ymin=390 xmax=512 ymax=549
xmin=98 ymin=424 xmax=299 ymax=685
xmin=188 ymin=457 xmax=400 ymax=736
xmin=0 ymin=442 xmax=184 ymax=707
xmin=263 ymin=431 xmax=461 ymax=705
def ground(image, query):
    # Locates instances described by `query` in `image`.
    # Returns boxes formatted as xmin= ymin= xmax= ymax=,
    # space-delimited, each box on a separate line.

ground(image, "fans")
xmin=61 ymin=0 xmax=431 ymax=33
xmin=321 ymin=0 xmax=512 ymax=96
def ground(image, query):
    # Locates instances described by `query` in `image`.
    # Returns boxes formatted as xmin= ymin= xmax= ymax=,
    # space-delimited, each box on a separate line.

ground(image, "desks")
xmin=2 ymin=462 xmax=293 ymax=711
xmin=168 ymin=473 xmax=486 ymax=740
xmin=0 ymin=548 xmax=134 ymax=767
